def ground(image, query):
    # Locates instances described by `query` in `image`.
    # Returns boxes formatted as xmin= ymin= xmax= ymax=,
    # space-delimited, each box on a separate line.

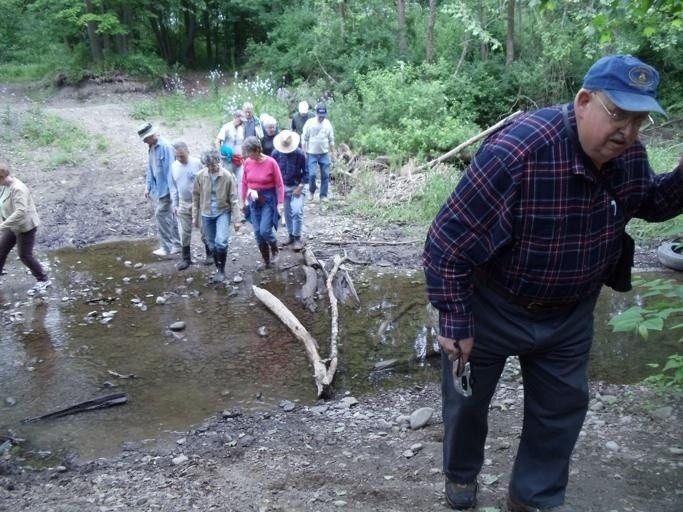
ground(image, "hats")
xmin=581 ymin=54 xmax=670 ymax=122
xmin=273 ymin=130 xmax=301 ymax=154
xmin=299 ymin=99 xmax=327 ymax=118
xmin=233 ymin=109 xmax=248 ymax=122
xmin=137 ymin=122 xmax=156 ymax=141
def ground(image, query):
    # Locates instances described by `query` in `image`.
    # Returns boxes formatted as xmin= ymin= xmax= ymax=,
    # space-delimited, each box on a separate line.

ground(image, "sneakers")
xmin=152 ymin=246 xmax=182 ymax=257
xmin=281 ymin=234 xmax=306 ymax=251
xmin=33 ymin=278 xmax=52 ymax=291
xmin=506 ymin=494 xmax=560 ymax=512
xmin=308 ymin=191 xmax=329 ymax=203
xmin=444 ymin=475 xmax=478 ymax=510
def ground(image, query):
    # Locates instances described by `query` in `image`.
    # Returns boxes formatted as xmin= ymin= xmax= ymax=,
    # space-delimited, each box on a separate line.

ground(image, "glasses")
xmin=592 ymin=92 xmax=655 ymax=133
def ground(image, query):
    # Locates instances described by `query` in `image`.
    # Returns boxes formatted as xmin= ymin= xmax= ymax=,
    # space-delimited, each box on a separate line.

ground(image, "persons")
xmin=166 ymin=98 xmax=336 ymax=285
xmin=1 ymin=166 xmax=51 ymax=293
xmin=142 ymin=133 xmax=182 ymax=256
xmin=422 ymin=53 xmax=683 ymax=510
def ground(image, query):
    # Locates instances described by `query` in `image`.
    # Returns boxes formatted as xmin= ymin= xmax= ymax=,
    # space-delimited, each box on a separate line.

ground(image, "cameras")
xmin=452 ymin=360 xmax=474 ymax=397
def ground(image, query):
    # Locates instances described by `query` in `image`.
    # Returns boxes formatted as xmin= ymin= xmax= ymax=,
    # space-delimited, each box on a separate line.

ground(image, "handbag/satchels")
xmin=603 ymin=230 xmax=635 ymax=293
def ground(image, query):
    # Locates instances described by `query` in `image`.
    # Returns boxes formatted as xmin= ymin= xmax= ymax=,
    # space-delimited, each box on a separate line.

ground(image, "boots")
xmin=178 ymin=246 xmax=192 ymax=270
xmin=202 ymin=243 xmax=215 ymax=265
xmin=257 ymin=241 xmax=280 ymax=272
xmin=211 ymin=253 xmax=226 ymax=283
